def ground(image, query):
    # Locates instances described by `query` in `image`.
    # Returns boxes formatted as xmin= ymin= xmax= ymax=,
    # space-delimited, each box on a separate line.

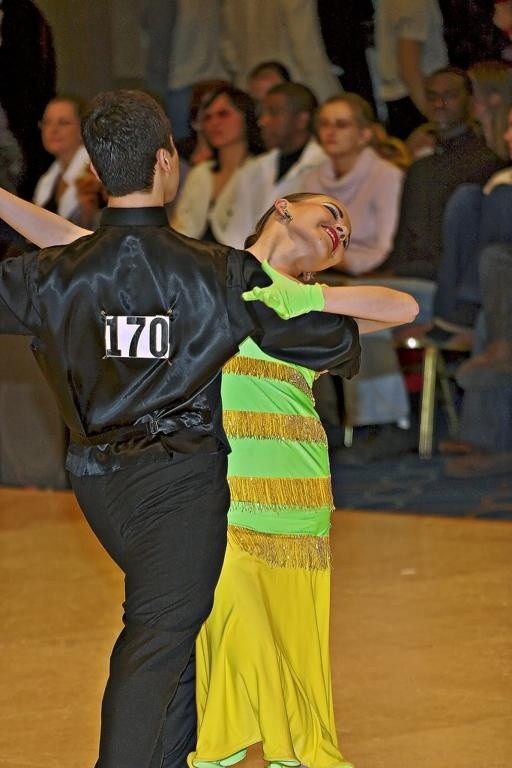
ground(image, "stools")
xmin=340 ymin=315 xmax=464 ymax=460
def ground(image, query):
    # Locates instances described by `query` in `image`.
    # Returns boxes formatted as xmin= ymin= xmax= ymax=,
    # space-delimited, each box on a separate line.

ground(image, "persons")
xmin=0 ymin=179 xmax=424 ymax=766
xmin=0 ymin=0 xmax=512 ymax=479
xmin=0 ymin=86 xmax=362 ymax=768
